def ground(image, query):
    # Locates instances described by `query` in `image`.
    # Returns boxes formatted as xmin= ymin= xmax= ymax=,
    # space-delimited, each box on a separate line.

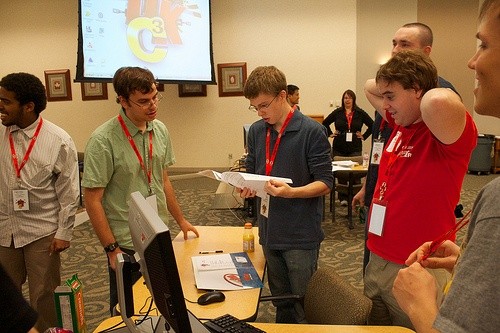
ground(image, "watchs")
xmin=104 ymin=242 xmax=119 ymax=253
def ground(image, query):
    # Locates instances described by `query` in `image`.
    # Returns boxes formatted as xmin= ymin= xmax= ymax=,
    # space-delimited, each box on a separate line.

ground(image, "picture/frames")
xmin=156 ymin=84 xmax=165 ymax=91
xmin=217 ymin=62 xmax=247 ymax=97
xmin=81 ymin=82 xmax=108 ymax=101
xmin=44 ymin=69 xmax=72 ymax=102
xmin=178 ymin=83 xmax=207 ymax=98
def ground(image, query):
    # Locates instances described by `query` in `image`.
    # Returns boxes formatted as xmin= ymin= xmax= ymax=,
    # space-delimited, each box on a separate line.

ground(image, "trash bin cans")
xmin=466 ymin=134 xmax=495 ymax=175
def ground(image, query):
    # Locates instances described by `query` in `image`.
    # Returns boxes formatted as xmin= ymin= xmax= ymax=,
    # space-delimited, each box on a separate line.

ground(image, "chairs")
xmin=259 ymin=266 xmax=373 ymax=326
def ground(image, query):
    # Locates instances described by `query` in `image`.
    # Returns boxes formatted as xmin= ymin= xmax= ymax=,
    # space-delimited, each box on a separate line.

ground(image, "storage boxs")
xmin=54 ymin=273 xmax=85 ymax=333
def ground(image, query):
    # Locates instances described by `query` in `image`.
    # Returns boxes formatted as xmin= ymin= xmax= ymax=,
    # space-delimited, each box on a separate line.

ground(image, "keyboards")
xmin=206 ymin=314 xmax=266 ymax=333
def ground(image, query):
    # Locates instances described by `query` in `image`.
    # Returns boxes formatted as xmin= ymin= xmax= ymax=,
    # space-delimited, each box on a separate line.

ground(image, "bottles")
xmin=363 ymin=152 xmax=369 ymax=168
xmin=243 ymin=223 xmax=254 ymax=252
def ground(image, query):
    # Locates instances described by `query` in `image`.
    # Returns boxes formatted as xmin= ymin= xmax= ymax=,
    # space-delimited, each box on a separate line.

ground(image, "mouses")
xmin=197 ymin=291 xmax=226 ymax=305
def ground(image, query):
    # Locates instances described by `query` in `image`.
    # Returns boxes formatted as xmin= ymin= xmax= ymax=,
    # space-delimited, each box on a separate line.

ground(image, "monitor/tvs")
xmin=116 ymin=191 xmax=192 ymax=333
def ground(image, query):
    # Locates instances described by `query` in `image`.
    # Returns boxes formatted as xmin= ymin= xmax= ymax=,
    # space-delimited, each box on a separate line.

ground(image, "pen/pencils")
xmin=199 ymin=250 xmax=223 ymax=254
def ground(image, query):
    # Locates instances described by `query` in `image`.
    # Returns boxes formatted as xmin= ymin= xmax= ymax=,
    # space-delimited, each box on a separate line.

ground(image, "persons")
xmin=0 ymin=72 xmax=80 ymax=333
xmin=322 ymin=90 xmax=375 ymax=207
xmin=287 ymin=85 xmax=300 ymax=111
xmin=236 ymin=66 xmax=334 ymax=324
xmin=81 ymin=67 xmax=199 ymax=318
xmin=364 ymin=48 xmax=476 ymax=332
xmin=352 ymin=23 xmax=462 ymax=280
xmin=0 ymin=261 xmax=39 ymax=333
xmin=392 ymin=0 xmax=500 ymax=333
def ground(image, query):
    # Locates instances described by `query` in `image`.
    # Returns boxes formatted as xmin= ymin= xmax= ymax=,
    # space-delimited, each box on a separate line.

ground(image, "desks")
xmin=331 ymin=171 xmax=367 ymax=229
xmin=93 ymin=316 xmax=417 ymax=333
xmin=115 ymin=226 xmax=267 ymax=321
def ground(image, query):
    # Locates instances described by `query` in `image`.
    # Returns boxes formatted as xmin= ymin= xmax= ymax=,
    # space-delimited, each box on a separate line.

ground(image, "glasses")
xmin=128 ymin=91 xmax=163 ymax=110
xmin=249 ymin=91 xmax=281 ymax=112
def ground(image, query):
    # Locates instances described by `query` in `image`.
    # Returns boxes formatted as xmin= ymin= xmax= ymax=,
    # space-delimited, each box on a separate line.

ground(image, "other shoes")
xmin=341 ymin=200 xmax=348 ymax=206
